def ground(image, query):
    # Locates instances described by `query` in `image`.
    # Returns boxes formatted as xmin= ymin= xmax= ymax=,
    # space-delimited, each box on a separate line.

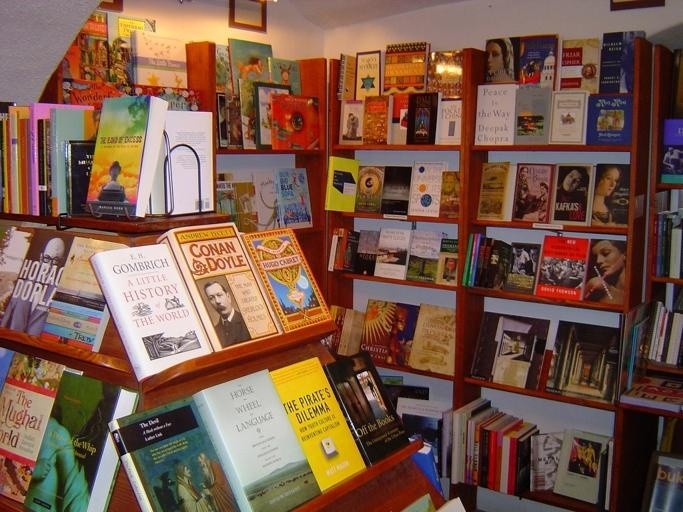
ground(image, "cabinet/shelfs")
xmin=44 ymin=38 xmax=329 ymax=305
xmin=616 ymin=44 xmax=682 ymax=512
xmin=460 ymin=34 xmax=654 ymax=511
xmin=325 ymin=47 xmax=467 ymax=511
xmin=1 ymin=211 xmax=450 ymax=511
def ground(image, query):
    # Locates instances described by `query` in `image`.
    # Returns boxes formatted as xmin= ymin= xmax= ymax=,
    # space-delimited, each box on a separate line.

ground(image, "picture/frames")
xmin=97 ymin=0 xmax=124 ymax=12
xmin=607 ymin=0 xmax=666 ymax=12
xmin=228 ymin=0 xmax=269 ymax=33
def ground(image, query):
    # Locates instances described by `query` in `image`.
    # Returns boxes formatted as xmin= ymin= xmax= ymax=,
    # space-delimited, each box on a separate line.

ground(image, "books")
xmin=452 ymin=31 xmax=643 ymax=500
xmin=2 ymin=98 xmax=467 ymax=512
xmin=619 ymin=117 xmax=683 ymax=511
xmin=40 ymin=13 xmax=462 ymax=512
